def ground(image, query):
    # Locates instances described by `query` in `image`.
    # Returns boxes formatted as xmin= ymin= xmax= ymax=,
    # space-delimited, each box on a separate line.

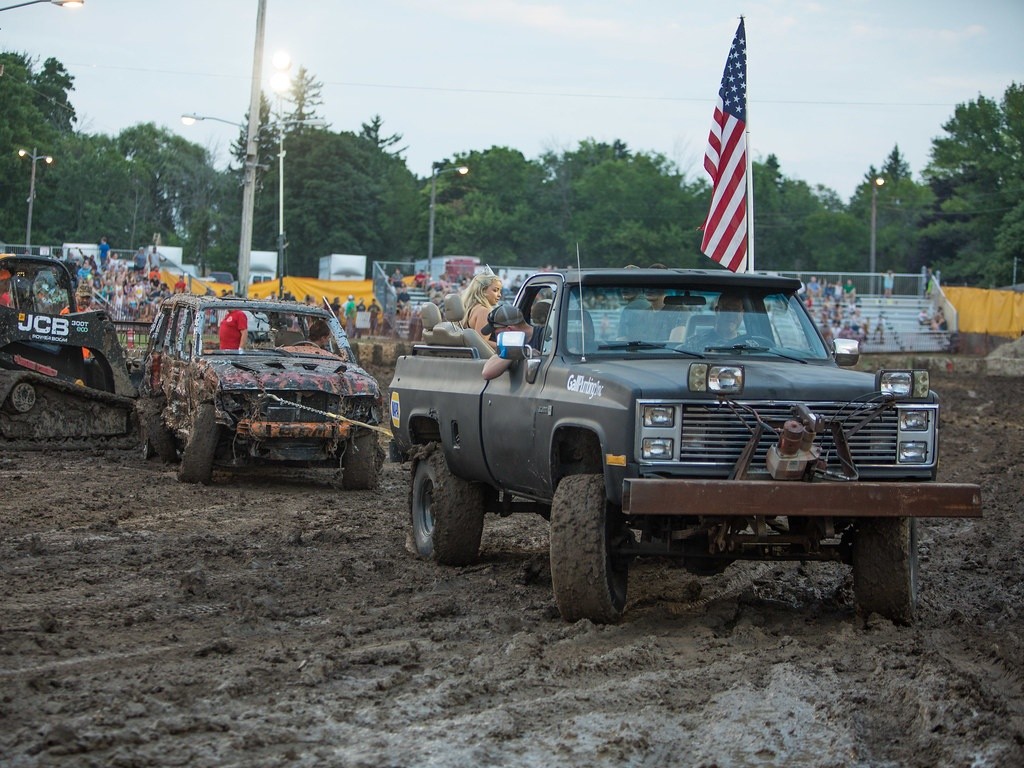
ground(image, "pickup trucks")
xmin=389 ymin=271 xmax=982 ymax=624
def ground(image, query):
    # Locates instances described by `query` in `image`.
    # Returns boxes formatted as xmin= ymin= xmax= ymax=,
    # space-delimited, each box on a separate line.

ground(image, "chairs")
xmin=275 ymin=332 xmax=305 ymax=347
xmin=685 ymin=315 xmax=719 ymax=340
xmin=566 ymin=310 xmax=601 ymax=353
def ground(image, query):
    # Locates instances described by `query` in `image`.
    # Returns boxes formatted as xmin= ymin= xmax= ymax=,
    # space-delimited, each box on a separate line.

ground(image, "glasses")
xmin=487 ymin=305 xmax=508 ymax=329
xmin=718 ymin=307 xmax=744 ymax=314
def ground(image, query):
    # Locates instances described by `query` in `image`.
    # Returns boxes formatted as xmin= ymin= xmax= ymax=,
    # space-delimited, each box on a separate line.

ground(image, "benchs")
xmin=378 ymin=287 xmax=948 ymax=358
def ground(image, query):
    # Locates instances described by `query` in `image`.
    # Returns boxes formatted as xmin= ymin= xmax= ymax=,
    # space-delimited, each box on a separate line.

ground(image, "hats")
xmin=481 ymin=304 xmax=523 ymax=335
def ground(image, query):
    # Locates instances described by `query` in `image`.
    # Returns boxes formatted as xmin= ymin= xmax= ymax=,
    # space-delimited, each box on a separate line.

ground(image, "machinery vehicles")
xmin=0 ymin=254 xmax=142 ymax=452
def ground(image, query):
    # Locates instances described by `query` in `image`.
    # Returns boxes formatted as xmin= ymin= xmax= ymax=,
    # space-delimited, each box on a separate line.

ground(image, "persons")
xmin=218 ymin=295 xmax=248 ymax=351
xmin=480 ymin=304 xmax=580 ymax=380
xmin=461 ymin=274 xmax=503 ymax=350
xmin=23 ymin=250 xmax=95 ymax=358
xmin=682 ymin=289 xmax=774 ymax=353
xmin=265 ymin=290 xmax=329 ymax=331
xmin=296 ymin=319 xmax=330 ymax=350
xmin=204 ymin=287 xmax=261 ymax=329
xmin=509 ymin=264 xmax=573 ymax=295
xmin=93 ymin=237 xmax=186 ymax=321
xmin=918 ymin=306 xmax=948 ymax=331
xmin=637 ymin=264 xmax=692 ymax=341
xmin=923 ymin=269 xmax=935 ymax=299
xmin=574 ymin=286 xmax=623 ymax=339
xmin=377 ymin=267 xmax=473 ymax=320
xmin=797 ymin=276 xmax=885 ymax=343
xmin=617 ymin=265 xmax=650 ymax=341
xmin=881 ymin=270 xmax=894 ymax=298
xmin=329 ymin=294 xmax=379 ymax=340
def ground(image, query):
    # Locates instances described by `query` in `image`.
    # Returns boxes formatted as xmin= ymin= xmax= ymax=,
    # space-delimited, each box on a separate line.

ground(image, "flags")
xmin=701 ymin=20 xmax=747 ymax=274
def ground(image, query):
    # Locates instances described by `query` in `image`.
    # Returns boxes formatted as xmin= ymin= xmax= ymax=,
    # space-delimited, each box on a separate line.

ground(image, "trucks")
xmin=248 ymin=251 xmax=278 ymax=290
xmin=318 ymin=254 xmax=366 ymax=281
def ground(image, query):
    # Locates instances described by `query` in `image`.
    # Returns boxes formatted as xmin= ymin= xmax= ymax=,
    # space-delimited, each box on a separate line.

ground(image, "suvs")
xmin=137 ymin=295 xmax=382 ymax=489
xmin=207 ymin=272 xmax=234 ymax=285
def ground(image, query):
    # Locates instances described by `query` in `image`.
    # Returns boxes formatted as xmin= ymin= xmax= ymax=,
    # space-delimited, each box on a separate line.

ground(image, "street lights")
xmin=426 ymin=167 xmax=469 ymax=277
xmin=870 ymin=177 xmax=884 ymax=293
xmin=180 ymin=112 xmax=332 ymax=299
xmin=19 ymin=147 xmax=53 ymax=247
